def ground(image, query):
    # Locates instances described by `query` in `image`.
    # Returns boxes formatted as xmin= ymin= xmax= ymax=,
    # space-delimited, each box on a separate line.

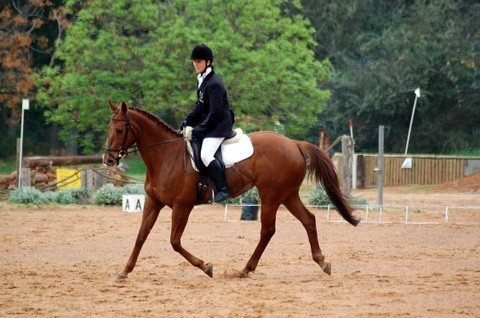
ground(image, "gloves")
xmin=181 ymin=120 xmax=193 ymax=130
xmin=192 ymin=126 xmax=206 ymax=139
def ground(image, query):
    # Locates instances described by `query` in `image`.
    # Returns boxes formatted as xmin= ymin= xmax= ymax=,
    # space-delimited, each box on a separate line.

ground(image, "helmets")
xmin=190 ymin=44 xmax=214 ymax=61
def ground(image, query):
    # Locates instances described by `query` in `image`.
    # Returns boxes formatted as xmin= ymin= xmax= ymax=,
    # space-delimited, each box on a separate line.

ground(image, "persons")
xmin=178 ymin=44 xmax=237 ymax=202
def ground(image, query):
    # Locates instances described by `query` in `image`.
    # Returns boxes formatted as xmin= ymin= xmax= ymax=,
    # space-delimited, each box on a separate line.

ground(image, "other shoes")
xmin=214 ymin=186 xmax=230 ymax=202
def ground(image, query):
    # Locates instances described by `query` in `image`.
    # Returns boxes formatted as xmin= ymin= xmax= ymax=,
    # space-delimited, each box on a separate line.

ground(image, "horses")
xmin=103 ymin=101 xmax=362 ymax=289
